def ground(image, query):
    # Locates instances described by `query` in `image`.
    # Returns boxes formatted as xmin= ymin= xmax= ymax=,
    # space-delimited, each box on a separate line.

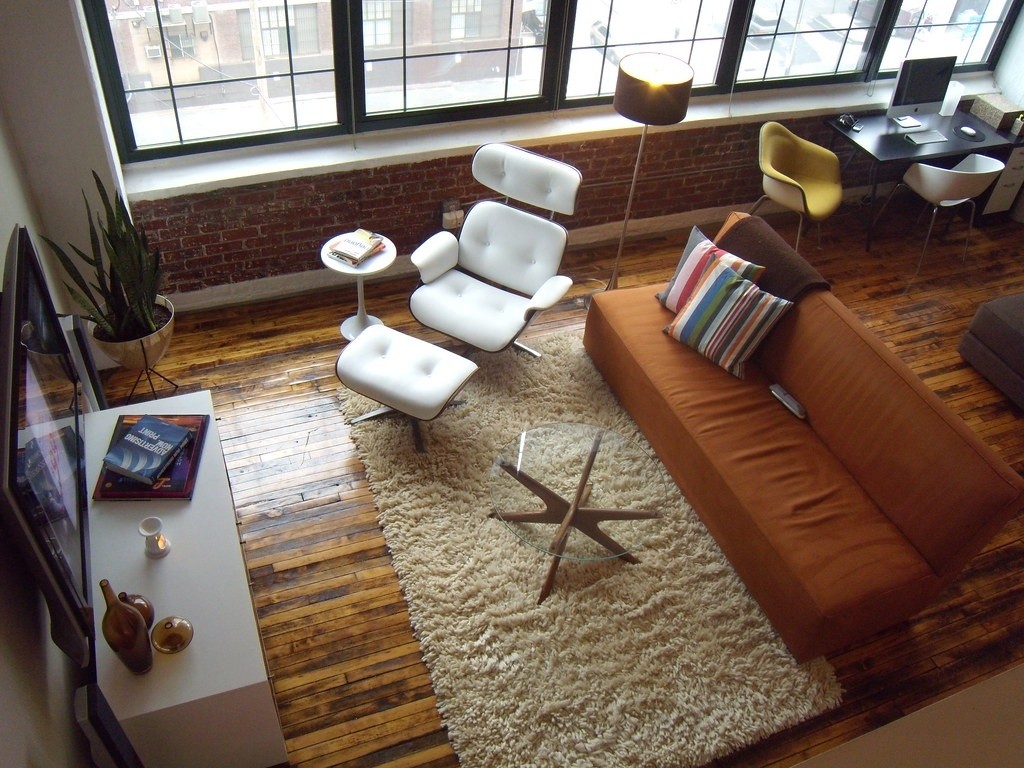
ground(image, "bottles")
xmin=150 ymin=615 xmax=194 ymax=655
xmin=98 ymin=578 xmax=153 ymax=676
xmin=118 ymin=591 xmax=154 ymax=632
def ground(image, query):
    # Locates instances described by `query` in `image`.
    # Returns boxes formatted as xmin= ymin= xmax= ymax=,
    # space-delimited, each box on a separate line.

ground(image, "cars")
xmin=589 ymin=16 xmax=624 ymax=64
xmin=521 ymin=9 xmax=545 ymax=32
xmin=746 ymin=10 xmax=779 ymax=42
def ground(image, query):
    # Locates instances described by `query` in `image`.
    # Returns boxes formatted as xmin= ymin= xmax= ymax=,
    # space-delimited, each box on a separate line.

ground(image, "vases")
xmin=99 ymin=579 xmax=193 ymax=675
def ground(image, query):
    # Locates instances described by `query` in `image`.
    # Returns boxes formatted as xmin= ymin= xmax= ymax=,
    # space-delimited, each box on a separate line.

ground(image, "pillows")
xmin=654 ymin=225 xmax=765 ymax=316
xmin=661 ymin=252 xmax=794 ymax=381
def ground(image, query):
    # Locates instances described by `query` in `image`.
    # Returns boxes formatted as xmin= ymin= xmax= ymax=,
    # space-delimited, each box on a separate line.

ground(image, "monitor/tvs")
xmin=0 ymin=224 xmax=94 ymax=670
xmin=886 ymin=56 xmax=957 ymax=127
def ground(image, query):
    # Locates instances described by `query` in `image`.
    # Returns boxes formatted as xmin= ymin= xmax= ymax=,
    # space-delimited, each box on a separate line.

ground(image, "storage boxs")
xmin=970 ymin=93 xmax=1024 ymax=137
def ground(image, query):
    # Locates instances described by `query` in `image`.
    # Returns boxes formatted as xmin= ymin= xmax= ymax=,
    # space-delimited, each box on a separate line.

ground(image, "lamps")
xmin=585 ymin=52 xmax=695 ymax=312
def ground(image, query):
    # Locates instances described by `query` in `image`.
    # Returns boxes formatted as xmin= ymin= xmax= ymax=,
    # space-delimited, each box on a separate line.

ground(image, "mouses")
xmin=961 ymin=127 xmax=976 ymax=136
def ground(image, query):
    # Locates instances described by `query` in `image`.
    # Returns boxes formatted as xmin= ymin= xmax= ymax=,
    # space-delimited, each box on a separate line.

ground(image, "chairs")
xmin=748 ymin=121 xmax=842 ymax=252
xmin=863 ymin=153 xmax=1005 ymax=275
xmin=408 ymin=142 xmax=583 ymax=358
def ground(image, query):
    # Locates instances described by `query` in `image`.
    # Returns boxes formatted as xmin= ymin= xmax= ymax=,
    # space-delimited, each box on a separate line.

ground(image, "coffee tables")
xmin=488 ymin=422 xmax=667 ymax=604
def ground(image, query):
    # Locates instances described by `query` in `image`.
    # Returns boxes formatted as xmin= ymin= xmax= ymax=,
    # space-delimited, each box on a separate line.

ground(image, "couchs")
xmin=581 ymin=209 xmax=1024 ymax=664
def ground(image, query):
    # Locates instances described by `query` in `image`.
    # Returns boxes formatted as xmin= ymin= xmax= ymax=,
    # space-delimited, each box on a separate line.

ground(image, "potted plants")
xmin=39 ymin=169 xmax=177 ymax=369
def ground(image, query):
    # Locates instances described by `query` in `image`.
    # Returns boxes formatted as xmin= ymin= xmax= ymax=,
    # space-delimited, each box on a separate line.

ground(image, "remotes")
xmin=769 ymin=384 xmax=805 ymax=419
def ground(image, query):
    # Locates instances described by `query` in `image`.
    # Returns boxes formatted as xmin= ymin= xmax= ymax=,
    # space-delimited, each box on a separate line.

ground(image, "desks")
xmin=824 ymin=107 xmax=1011 ymax=251
xmin=321 ymin=233 xmax=397 ymax=341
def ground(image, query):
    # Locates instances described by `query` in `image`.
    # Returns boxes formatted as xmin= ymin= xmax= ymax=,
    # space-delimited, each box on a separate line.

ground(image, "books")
xmin=92 ymin=414 xmax=210 ymax=503
xmin=103 ymin=415 xmax=193 ymax=485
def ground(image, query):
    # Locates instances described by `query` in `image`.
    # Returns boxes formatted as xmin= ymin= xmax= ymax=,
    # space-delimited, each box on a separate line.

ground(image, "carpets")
xmin=335 ymin=327 xmax=848 ymax=768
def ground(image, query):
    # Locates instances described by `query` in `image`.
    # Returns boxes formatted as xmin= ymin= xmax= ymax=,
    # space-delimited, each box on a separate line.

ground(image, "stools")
xmin=335 ymin=325 xmax=480 ymax=452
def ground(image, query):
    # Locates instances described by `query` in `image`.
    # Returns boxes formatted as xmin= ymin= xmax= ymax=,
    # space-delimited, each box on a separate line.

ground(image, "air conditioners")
xmin=145 ymin=46 xmax=162 ymax=59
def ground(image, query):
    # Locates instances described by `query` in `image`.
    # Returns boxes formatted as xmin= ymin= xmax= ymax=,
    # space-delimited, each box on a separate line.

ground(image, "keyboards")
xmin=905 ymin=129 xmax=949 ymax=145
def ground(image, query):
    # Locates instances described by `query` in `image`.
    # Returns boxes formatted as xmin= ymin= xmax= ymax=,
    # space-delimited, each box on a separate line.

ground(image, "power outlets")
xmin=444 ymin=198 xmax=461 ymax=213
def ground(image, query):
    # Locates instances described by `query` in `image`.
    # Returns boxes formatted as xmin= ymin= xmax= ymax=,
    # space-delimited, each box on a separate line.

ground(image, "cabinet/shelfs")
xmin=958 ymin=107 xmax=1024 ymax=228
xmin=74 ymin=389 xmax=289 ymax=768
